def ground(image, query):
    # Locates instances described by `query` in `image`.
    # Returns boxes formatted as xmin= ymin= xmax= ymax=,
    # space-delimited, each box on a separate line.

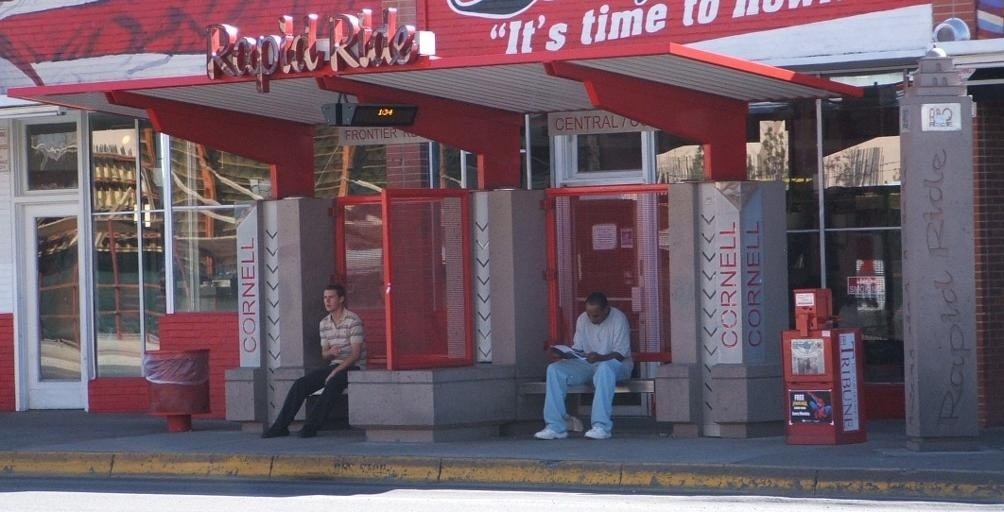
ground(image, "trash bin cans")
xmin=144 ymin=349 xmax=212 ymax=432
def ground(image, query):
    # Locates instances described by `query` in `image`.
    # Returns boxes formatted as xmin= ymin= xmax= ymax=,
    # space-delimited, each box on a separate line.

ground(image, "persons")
xmin=535 ymin=291 xmax=633 ymax=439
xmin=262 ymin=284 xmax=367 ymax=437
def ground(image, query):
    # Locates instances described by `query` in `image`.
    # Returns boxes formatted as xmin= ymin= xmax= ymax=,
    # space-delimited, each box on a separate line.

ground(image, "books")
xmin=551 ymin=344 xmax=589 ymax=361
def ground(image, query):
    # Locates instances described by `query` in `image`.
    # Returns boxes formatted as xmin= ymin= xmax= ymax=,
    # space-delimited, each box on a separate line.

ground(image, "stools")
xmin=311 ymin=387 xmax=347 ymax=397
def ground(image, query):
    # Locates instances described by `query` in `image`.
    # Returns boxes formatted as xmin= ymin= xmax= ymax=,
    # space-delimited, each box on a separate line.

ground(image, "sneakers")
xmin=535 ymin=427 xmax=568 ymax=440
xmin=263 ymin=425 xmax=290 ymax=438
xmin=297 ymin=425 xmax=317 ymax=437
xmin=584 ymin=427 xmax=612 ymax=440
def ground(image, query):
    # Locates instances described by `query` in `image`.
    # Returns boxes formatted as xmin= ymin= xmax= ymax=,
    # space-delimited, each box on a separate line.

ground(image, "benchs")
xmin=525 ymin=375 xmax=654 ymax=395
